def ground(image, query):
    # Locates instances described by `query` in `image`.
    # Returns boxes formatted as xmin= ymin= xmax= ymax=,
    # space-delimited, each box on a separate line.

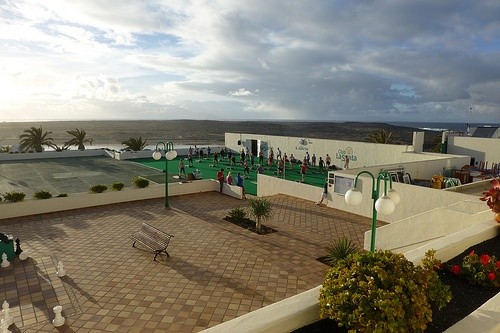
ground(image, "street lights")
xmin=152 ymin=141 xmax=178 ymax=210
xmin=345 ymin=169 xmax=401 ymax=254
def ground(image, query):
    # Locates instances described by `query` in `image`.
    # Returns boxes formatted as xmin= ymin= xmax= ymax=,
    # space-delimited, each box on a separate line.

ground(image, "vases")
xmin=494 ymin=213 xmax=500 ymax=223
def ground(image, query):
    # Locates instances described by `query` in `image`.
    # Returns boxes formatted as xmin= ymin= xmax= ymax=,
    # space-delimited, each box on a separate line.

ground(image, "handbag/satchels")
xmin=321 ymin=192 xmax=327 ymax=197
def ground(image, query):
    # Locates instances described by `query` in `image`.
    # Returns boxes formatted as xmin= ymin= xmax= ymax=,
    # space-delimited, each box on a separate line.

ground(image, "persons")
xmin=207 ymin=145 xmax=211 ymax=159
xmin=227 ymin=149 xmax=236 ymax=166
xmin=268 ymin=148 xmax=274 ymax=167
xmin=213 ymin=151 xmax=219 ymax=166
xmin=300 ymin=163 xmax=306 ymax=182
xmin=221 ymin=147 xmax=227 ymax=160
xmin=319 ymin=156 xmax=325 ymax=172
xmin=303 ymin=151 xmax=310 ymax=166
xmin=226 ymin=171 xmax=232 ymax=185
xmin=217 ymin=169 xmax=224 ymax=193
xmin=325 ymin=154 xmax=331 ymax=167
xmin=251 ymin=153 xmax=255 ymax=165
xmin=179 ymin=159 xmax=186 ymax=179
xmin=279 ymin=158 xmax=284 ymax=173
xmin=241 ymin=148 xmax=245 ymax=161
xmin=317 ymin=177 xmax=328 ymax=205
xmin=289 ymin=154 xmax=295 ymax=167
xmin=259 ymin=148 xmax=264 ymax=162
xmin=243 ymin=159 xmax=250 ymax=174
xmin=342 ymin=154 xmax=349 ymax=169
xmin=283 ymin=153 xmax=287 ymax=161
xmin=194 ymin=144 xmax=204 ymax=159
xmin=277 ymin=147 xmax=281 ymax=161
xmin=235 ymin=172 xmax=246 ymax=200
xmin=188 ymin=145 xmax=193 ymax=162
xmin=312 ymin=154 xmax=316 ymax=166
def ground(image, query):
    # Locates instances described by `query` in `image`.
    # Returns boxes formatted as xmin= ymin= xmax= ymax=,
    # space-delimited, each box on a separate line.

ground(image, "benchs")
xmin=130 ymin=222 xmax=174 ymax=261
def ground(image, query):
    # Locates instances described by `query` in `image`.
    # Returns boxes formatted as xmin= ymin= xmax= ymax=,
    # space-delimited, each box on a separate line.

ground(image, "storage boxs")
xmin=0 ymin=235 xmax=15 ymax=262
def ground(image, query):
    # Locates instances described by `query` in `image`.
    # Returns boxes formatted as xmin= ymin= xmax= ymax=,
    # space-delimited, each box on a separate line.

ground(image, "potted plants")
xmin=90 ymin=175 xmax=150 ymax=193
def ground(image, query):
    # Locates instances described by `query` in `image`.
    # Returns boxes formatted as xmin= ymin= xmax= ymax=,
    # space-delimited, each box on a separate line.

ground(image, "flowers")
xmin=450 ymin=251 xmax=500 ymax=288
xmin=479 ymin=175 xmax=500 ymax=213
